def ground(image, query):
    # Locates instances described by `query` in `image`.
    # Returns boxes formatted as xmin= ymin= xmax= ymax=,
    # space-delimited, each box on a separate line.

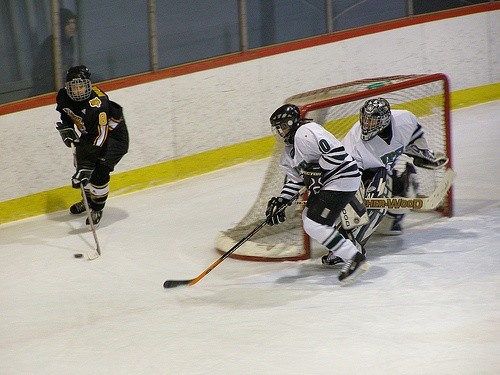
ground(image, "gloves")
xmin=302 ymin=168 xmax=325 ymax=194
xmin=386 ymin=152 xmax=415 ymax=179
xmin=265 ymin=196 xmax=292 ymax=226
xmin=71 ymin=161 xmax=96 ymax=188
xmin=56 ymin=122 xmax=80 ymax=148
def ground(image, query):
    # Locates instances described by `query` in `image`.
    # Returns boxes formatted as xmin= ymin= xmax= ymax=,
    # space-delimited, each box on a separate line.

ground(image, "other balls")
xmin=73 ymin=253 xmax=83 ymax=258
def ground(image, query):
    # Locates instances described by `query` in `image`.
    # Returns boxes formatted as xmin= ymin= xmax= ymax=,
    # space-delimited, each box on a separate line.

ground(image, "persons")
xmin=341 ymin=98 xmax=449 ymax=246
xmin=55 ymin=65 xmax=129 ymax=229
xmin=266 ymin=103 xmax=371 ymax=287
xmin=32 ymin=8 xmax=77 ymax=96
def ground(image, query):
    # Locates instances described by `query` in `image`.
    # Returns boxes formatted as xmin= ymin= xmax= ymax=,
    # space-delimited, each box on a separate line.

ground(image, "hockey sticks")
xmin=163 ymin=186 xmax=308 ymax=288
xmin=364 ymin=167 xmax=459 ymax=210
xmin=70 ymin=142 xmax=101 ymax=260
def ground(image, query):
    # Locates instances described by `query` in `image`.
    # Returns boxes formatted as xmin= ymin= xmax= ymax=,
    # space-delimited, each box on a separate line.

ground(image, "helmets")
xmin=65 ymin=64 xmax=91 ymax=100
xmin=360 ymin=97 xmax=391 ymax=141
xmin=269 ymin=104 xmax=301 ymax=146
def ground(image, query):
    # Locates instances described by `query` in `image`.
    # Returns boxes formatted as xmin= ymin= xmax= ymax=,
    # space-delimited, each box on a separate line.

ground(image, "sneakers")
xmin=337 ymin=252 xmax=369 ymax=284
xmin=321 ymin=250 xmax=343 ymax=266
xmin=70 ymin=202 xmax=85 ymax=214
xmin=86 ymin=210 xmax=102 ymax=231
